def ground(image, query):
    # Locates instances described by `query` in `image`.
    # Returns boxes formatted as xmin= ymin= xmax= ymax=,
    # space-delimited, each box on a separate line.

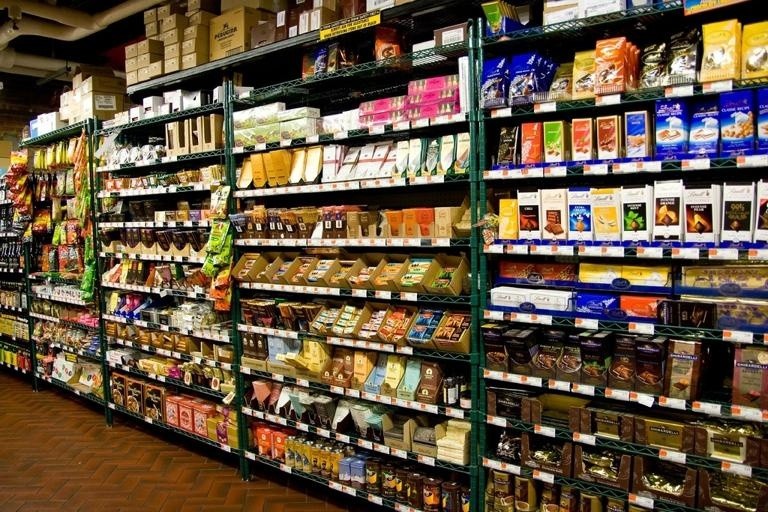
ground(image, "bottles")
xmin=0 ymin=205 xmax=31 ymax=376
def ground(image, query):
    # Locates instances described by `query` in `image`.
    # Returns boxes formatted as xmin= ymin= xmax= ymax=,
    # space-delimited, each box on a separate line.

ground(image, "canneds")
xmin=284 ymin=432 xmax=471 ymax=512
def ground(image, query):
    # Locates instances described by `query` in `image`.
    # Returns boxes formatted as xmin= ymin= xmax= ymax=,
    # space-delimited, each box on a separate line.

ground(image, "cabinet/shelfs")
xmin=0 ymin=0 xmax=767 ymax=510
